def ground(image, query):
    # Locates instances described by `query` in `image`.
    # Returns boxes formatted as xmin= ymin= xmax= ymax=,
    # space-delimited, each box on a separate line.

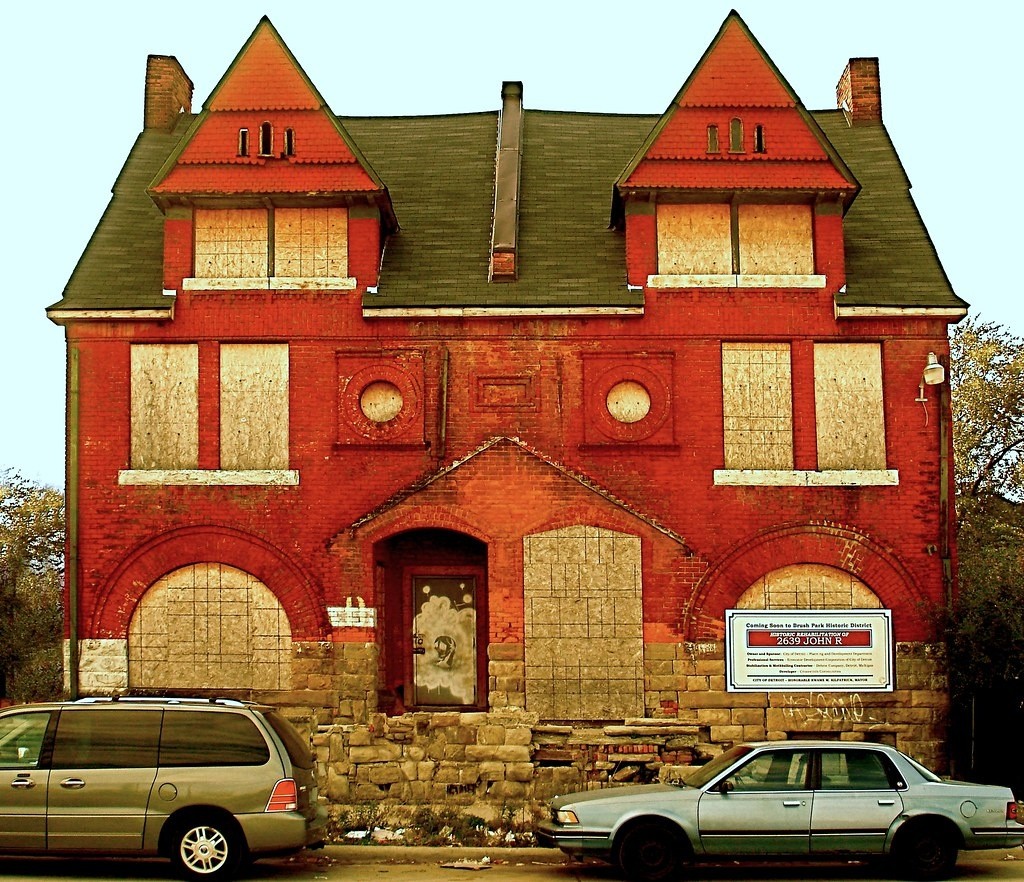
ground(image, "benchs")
xmin=848 ymin=771 xmax=884 ymax=784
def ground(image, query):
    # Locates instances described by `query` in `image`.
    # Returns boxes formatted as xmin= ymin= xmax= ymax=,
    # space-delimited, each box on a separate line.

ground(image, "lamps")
xmin=915 ymin=352 xmax=945 ymax=401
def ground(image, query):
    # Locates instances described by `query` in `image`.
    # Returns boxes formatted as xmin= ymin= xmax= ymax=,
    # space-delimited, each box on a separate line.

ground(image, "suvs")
xmin=1 ymin=693 xmax=335 ymax=882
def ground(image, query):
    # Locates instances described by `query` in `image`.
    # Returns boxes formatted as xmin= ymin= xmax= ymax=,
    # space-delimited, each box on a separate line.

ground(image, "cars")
xmin=535 ymin=740 xmax=1024 ymax=882
